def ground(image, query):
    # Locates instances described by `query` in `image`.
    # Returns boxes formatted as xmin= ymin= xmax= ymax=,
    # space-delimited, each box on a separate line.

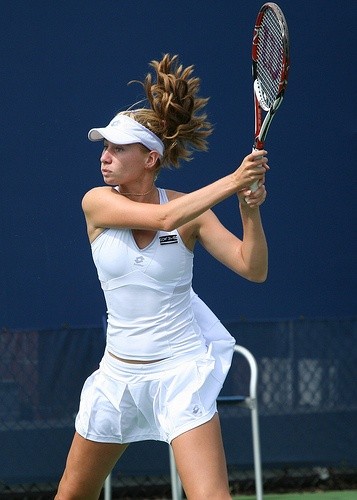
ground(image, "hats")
xmin=87 ymin=113 xmax=165 ymax=162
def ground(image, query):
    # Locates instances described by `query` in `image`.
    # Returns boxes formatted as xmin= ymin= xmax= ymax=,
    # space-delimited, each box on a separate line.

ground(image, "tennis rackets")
xmin=251 ymin=2 xmax=289 ymax=194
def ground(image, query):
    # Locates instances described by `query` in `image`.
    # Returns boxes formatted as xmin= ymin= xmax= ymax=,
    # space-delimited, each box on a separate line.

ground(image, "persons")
xmin=46 ymin=53 xmax=270 ymax=500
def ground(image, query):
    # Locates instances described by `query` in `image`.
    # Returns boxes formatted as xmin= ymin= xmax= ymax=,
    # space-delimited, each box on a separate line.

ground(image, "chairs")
xmin=105 ymin=344 xmax=264 ymax=500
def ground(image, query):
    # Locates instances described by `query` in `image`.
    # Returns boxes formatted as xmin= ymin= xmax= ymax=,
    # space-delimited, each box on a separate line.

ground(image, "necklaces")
xmin=116 ymin=182 xmax=155 ymax=196
